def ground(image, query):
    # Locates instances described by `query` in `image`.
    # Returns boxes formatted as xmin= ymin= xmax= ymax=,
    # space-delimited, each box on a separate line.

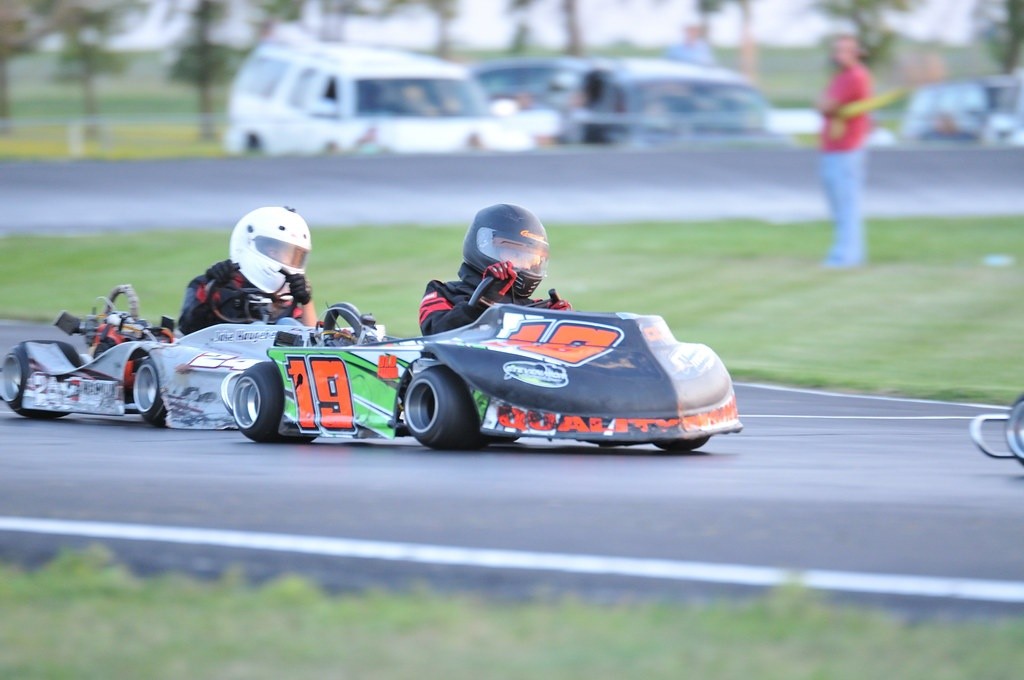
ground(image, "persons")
xmin=818 ymin=34 xmax=878 ymax=272
xmin=416 ymin=201 xmax=577 ymax=342
xmin=176 ymin=207 xmax=322 ymax=340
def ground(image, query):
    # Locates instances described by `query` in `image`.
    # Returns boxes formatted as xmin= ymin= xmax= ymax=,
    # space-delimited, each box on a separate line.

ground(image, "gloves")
xmin=547 ymin=300 xmax=571 ymax=311
xmin=482 ymin=261 xmax=517 ymax=303
xmin=286 ymin=273 xmax=312 ymax=305
xmin=207 ymin=259 xmax=237 ymax=286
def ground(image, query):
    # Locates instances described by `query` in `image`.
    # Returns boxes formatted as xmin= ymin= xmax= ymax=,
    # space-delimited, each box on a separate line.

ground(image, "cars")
xmin=904 ymin=77 xmax=1023 ymax=142
xmin=469 ymin=56 xmax=787 ymax=148
xmin=227 ymin=36 xmax=537 ymax=153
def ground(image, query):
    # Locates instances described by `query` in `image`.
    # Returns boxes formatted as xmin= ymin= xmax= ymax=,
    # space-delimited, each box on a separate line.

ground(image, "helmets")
xmin=461 ymin=204 xmax=550 ymax=297
xmin=229 ymin=207 xmax=312 ymax=293
